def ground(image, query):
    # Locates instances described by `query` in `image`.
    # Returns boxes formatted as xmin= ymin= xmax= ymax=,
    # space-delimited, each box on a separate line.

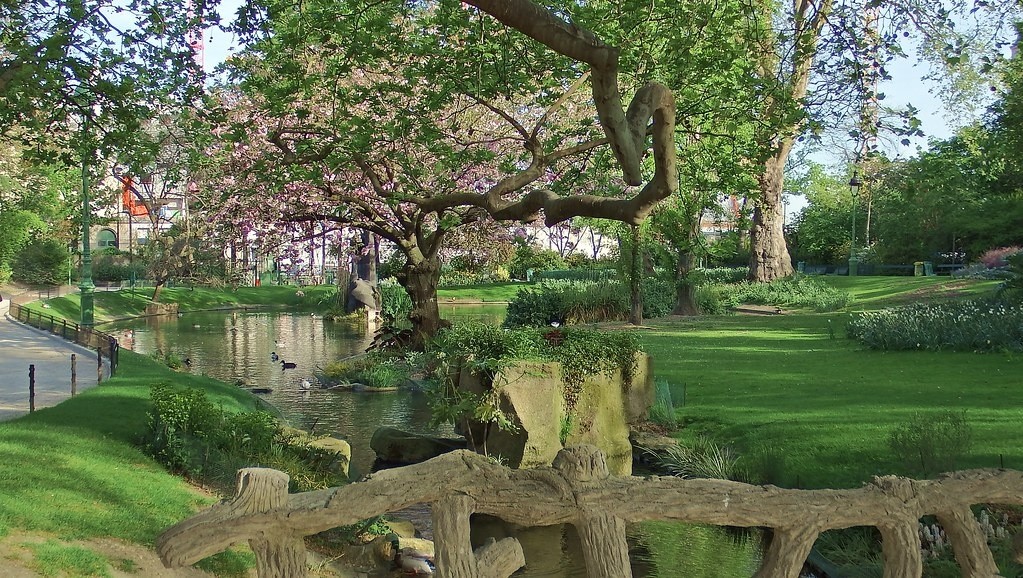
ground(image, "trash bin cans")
xmin=914 ymin=262 xmax=932 ymax=276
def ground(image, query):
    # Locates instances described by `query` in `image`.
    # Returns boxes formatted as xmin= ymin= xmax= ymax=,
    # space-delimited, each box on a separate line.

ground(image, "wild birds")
xmin=192 ymin=323 xmax=202 ymax=328
xmin=177 ymin=310 xmax=184 ymax=318
xmin=300 ymin=376 xmax=311 ymax=390
xmin=181 ymin=358 xmax=192 ymax=368
xmin=274 ymin=340 xmax=287 ymax=348
xmin=271 ymin=351 xmax=297 ymax=368
xmin=310 ymin=312 xmax=321 ymax=319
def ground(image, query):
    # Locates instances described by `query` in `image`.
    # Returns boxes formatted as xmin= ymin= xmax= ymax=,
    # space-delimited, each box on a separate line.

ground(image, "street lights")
xmin=72 ymin=76 xmax=98 ymax=328
xmin=848 ymin=170 xmax=860 ymax=276
